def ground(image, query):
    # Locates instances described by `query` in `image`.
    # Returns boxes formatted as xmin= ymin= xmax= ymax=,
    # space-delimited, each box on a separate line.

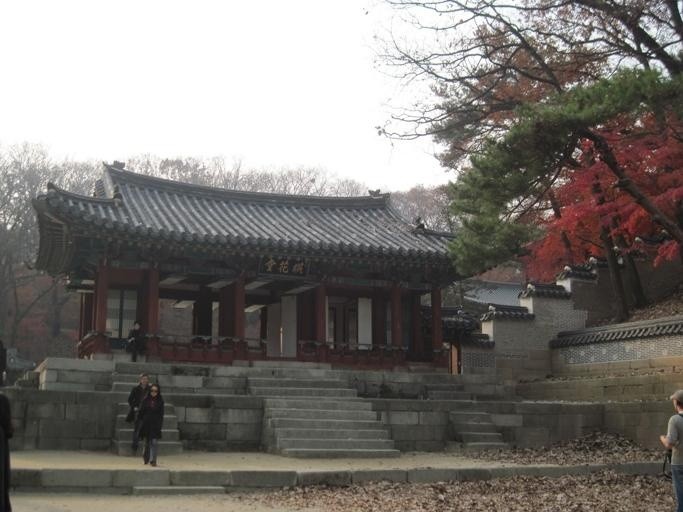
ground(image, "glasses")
xmin=150 ymin=390 xmax=158 ymax=393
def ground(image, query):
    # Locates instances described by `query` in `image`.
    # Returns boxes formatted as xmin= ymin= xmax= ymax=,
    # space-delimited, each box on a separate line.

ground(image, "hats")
xmin=669 ymin=390 xmax=683 ymax=404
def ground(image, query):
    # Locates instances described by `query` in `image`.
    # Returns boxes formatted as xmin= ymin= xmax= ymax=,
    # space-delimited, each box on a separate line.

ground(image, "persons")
xmin=127 ymin=321 xmax=146 ymax=363
xmin=658 ymin=390 xmax=683 ymax=511
xmin=127 ymin=373 xmax=150 ymax=452
xmin=1 ymin=392 xmax=15 ymax=512
xmin=0 ymin=341 xmax=7 ymax=391
xmin=135 ymin=383 xmax=164 ymax=466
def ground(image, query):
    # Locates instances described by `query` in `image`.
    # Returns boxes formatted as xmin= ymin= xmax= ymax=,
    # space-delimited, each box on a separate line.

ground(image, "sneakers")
xmin=133 ymin=445 xmax=157 ymax=466
